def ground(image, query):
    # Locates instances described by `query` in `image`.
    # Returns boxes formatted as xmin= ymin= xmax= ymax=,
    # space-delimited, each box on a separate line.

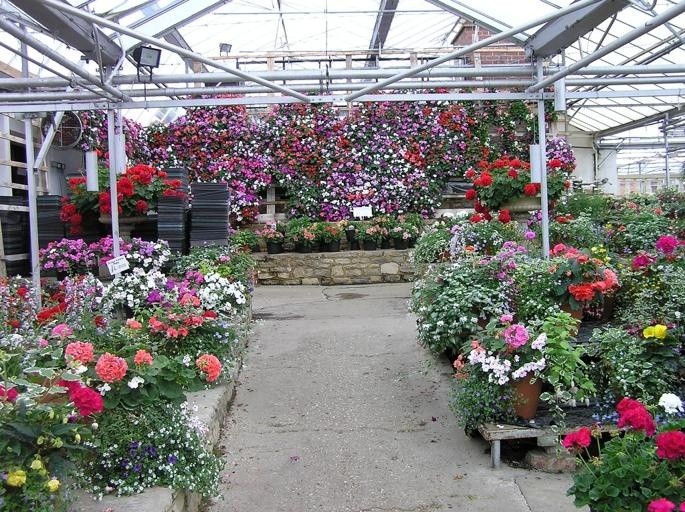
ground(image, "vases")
xmin=241 ymin=238 xmax=416 ymax=254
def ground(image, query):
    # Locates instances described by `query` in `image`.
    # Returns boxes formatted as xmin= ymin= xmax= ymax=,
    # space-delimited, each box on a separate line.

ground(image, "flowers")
xmin=403 ymin=187 xmax=684 ymax=512
xmin=1 ymin=234 xmax=258 ymax=512
xmin=228 ymin=213 xmax=426 ymax=246
xmin=60 ymin=149 xmax=187 ymax=239
xmin=464 ymin=137 xmax=577 ymax=208
